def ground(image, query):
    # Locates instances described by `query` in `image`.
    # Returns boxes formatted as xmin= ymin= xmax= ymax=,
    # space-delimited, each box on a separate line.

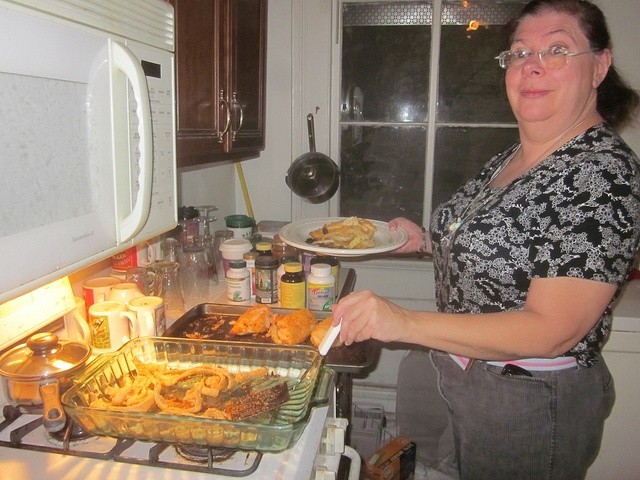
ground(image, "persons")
xmin=328 ymin=0 xmax=640 ymax=480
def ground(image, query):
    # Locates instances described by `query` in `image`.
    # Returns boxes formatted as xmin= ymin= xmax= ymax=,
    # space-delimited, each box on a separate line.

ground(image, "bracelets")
xmin=415 ymin=226 xmax=427 ymax=254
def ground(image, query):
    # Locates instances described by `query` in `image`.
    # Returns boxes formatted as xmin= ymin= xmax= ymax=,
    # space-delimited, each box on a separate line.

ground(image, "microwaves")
xmin=1 ymin=1 xmax=179 ymax=304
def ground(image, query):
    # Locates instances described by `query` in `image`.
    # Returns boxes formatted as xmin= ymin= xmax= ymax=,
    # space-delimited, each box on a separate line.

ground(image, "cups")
xmin=214 ymin=230 xmax=234 ymax=253
xmin=182 ymin=248 xmax=208 ymax=279
xmin=126 ymin=296 xmax=166 ymax=339
xmin=125 ymin=266 xmax=165 ymax=298
xmin=111 ymin=282 xmax=146 ymax=305
xmin=83 ymin=276 xmax=122 ymax=321
xmin=200 ymin=246 xmax=219 ymax=282
xmin=149 ymin=264 xmax=181 ymax=300
xmin=86 ymin=302 xmax=140 ymax=355
xmin=112 ymin=242 xmax=154 ymax=272
xmin=160 ymin=243 xmax=183 ymax=266
xmin=148 ymin=235 xmax=178 ymax=261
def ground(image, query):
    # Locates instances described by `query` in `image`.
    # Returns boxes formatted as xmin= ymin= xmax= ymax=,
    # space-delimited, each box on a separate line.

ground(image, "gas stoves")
xmin=1 ymin=361 xmax=351 ymax=479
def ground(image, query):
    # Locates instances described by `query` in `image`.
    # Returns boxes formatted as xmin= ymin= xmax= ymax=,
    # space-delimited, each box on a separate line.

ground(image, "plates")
xmin=277 ymin=215 xmax=410 ymax=258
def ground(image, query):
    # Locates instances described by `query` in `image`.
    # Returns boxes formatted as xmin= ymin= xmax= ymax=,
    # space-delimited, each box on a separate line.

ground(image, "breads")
xmin=268 ymin=308 xmax=316 ymax=344
xmin=310 ymin=316 xmax=342 ymax=349
xmin=228 ymin=303 xmax=271 ymax=335
xmin=309 ymin=217 xmax=376 ymax=249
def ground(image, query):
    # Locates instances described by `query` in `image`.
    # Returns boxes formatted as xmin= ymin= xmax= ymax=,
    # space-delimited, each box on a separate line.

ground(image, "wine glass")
xmin=195 ymin=206 xmax=219 ymax=217
xmin=193 ymin=217 xmax=218 ymax=246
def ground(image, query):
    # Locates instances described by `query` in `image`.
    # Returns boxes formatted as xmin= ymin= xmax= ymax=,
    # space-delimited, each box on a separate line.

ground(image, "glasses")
xmin=493 ymin=43 xmax=599 ymax=70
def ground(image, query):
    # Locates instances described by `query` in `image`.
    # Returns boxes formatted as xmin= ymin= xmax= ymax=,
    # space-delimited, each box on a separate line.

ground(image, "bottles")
xmin=255 ymin=241 xmax=273 ymax=256
xmin=245 ymin=251 xmax=262 ymax=299
xmin=277 ymin=261 xmax=307 ymax=309
xmin=225 ymin=260 xmax=250 ymax=302
xmin=221 ymin=237 xmax=253 ymax=263
xmin=277 ymin=255 xmax=290 ymax=301
xmin=306 ymin=263 xmax=337 ymax=310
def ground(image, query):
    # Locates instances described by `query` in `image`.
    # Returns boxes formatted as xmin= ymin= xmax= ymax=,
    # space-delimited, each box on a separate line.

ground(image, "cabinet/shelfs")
xmin=170 ymin=1 xmax=267 ymax=168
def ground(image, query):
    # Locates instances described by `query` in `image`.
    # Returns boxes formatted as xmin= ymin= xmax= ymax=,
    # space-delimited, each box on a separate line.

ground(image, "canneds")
xmin=254 ymin=256 xmax=280 ymax=304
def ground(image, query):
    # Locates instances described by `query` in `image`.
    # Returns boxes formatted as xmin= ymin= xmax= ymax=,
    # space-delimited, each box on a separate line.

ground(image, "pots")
xmin=284 ymin=113 xmax=340 ymax=204
xmin=0 ymin=332 xmax=92 ymax=433
